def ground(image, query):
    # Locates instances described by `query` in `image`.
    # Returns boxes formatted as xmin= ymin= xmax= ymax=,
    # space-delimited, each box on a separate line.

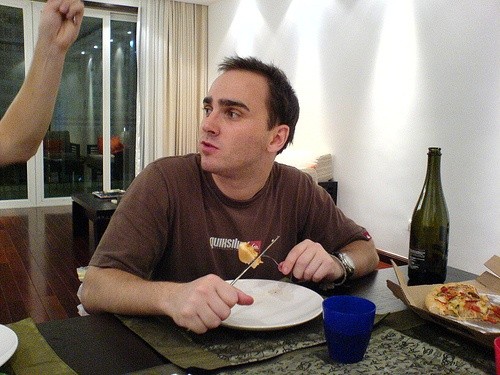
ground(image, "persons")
xmin=79 ymin=54 xmax=379 ymax=335
xmin=0 ymin=0 xmax=85 ymax=166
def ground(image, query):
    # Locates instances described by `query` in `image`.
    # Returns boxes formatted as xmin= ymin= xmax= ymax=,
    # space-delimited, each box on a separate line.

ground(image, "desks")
xmin=0 ymin=264 xmax=500 ymax=375
xmin=71 ymin=193 xmax=122 ymax=241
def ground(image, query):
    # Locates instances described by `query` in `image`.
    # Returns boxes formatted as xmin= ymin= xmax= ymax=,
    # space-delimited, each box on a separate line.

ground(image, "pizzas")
xmin=423 ymin=280 xmax=500 ymax=325
xmin=238 ymin=241 xmax=264 ymax=270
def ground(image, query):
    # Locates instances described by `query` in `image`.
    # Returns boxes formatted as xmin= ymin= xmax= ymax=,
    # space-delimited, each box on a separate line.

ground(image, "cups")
xmin=494 ymin=337 xmax=500 ymax=375
xmin=322 ymin=296 xmax=376 ymax=363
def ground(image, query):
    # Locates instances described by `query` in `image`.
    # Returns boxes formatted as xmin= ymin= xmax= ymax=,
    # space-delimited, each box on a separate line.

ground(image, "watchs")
xmin=328 ymin=251 xmax=356 ymax=287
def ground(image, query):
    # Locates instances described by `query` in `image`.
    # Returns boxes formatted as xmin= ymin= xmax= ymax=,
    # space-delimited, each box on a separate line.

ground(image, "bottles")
xmin=408 ymin=147 xmax=449 ymax=284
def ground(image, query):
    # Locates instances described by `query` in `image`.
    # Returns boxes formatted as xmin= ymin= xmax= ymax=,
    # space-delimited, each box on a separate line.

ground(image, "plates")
xmin=453 ymin=290 xmax=500 ymax=335
xmin=0 ymin=324 xmax=19 ymax=367
xmin=222 ymin=279 xmax=324 ymax=329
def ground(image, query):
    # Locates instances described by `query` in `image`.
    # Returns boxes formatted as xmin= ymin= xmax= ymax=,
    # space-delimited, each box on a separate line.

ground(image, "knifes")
xmin=230 ymin=236 xmax=280 ymax=286
